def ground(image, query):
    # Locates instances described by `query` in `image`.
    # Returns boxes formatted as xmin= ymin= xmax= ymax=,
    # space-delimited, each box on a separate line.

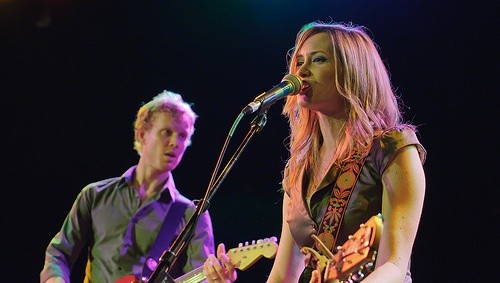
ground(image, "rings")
xmin=212 ymin=276 xmax=218 ymax=280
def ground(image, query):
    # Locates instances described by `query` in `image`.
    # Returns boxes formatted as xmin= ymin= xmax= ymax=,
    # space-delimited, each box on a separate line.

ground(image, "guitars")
xmin=114 ymin=237 xmax=278 ymax=282
xmin=299 ymin=213 xmax=385 ymax=283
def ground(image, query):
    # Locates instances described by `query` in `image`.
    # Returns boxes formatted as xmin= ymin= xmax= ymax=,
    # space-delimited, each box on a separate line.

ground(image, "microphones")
xmin=241 ymin=74 xmax=302 ymax=114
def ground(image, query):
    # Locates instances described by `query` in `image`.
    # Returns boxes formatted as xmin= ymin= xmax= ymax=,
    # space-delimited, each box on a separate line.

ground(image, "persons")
xmin=41 ymin=90 xmax=237 ymax=283
xmin=264 ymin=23 xmax=427 ymax=283
